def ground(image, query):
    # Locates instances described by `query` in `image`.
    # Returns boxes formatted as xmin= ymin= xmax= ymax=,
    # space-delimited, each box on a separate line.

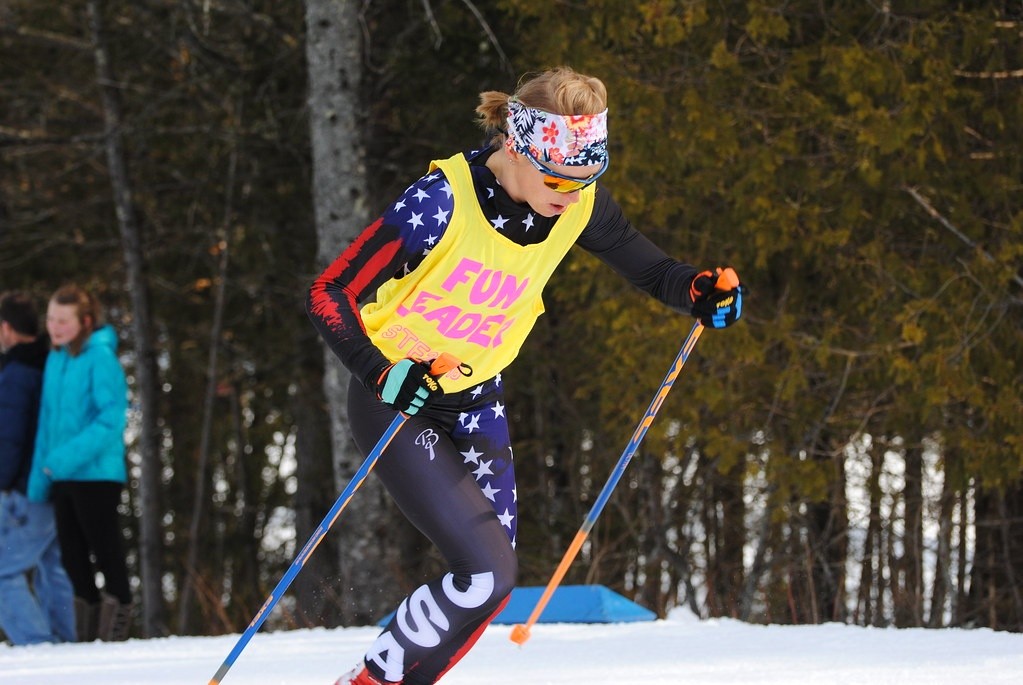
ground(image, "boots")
xmin=97 ymin=591 xmax=132 ymax=641
xmin=73 ymin=594 xmax=99 ymax=642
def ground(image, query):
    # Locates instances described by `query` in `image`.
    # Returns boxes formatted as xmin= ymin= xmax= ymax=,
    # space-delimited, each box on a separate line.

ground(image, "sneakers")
xmin=334 ymin=661 xmax=400 ymax=685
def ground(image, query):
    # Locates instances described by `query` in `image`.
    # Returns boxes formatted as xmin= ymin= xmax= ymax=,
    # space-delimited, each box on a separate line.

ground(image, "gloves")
xmin=377 ymin=357 xmax=444 ymax=416
xmin=689 ymin=267 xmax=742 ymax=330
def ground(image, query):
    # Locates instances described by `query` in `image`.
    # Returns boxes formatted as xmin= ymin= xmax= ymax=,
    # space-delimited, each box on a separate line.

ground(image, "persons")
xmin=27 ymin=285 xmax=133 ymax=642
xmin=0 ymin=291 xmax=76 ymax=646
xmin=306 ymin=69 xmax=742 ymax=685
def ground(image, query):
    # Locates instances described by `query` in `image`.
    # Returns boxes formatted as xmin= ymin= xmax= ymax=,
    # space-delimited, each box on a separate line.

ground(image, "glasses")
xmin=506 ymin=117 xmax=609 ymax=194
